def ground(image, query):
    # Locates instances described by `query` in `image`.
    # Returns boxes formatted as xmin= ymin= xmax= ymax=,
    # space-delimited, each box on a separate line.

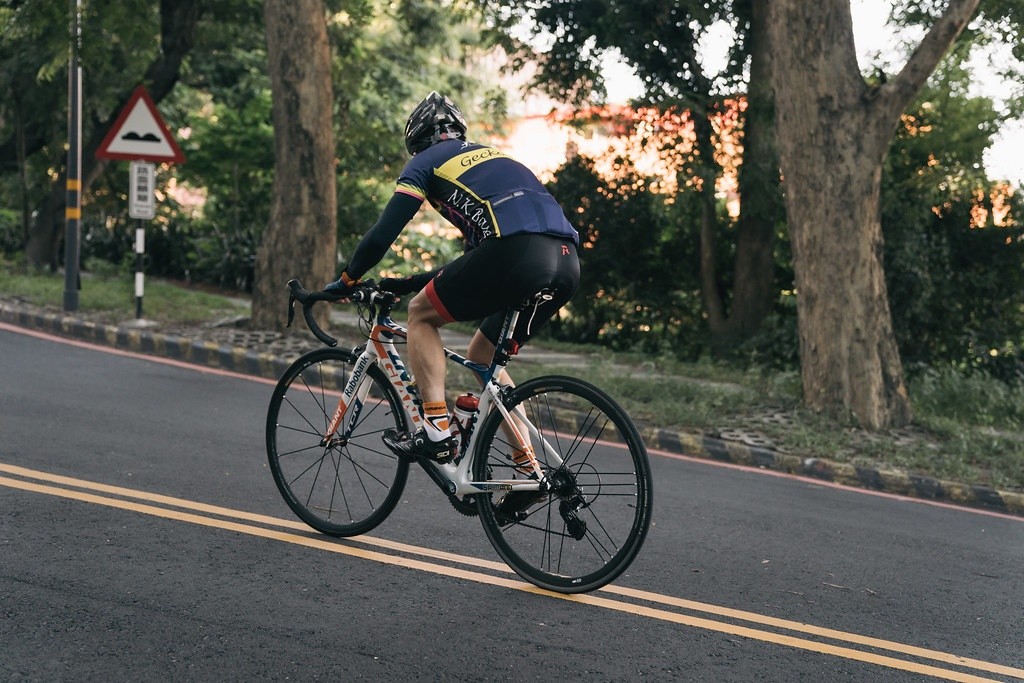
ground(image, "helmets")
xmin=404 ymin=91 xmax=468 ymax=156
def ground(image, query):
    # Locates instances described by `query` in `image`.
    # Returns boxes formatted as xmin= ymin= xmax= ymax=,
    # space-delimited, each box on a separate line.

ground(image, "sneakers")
xmin=493 ymin=471 xmax=547 ymax=521
xmin=380 ymin=427 xmax=458 ymax=463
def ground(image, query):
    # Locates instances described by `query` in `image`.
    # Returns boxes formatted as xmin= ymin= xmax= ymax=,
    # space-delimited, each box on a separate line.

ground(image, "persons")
xmin=322 ymin=90 xmax=582 ymax=526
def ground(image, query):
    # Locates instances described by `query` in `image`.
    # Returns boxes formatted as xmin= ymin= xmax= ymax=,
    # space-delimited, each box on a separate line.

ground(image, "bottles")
xmin=446 ymin=392 xmax=479 ymax=460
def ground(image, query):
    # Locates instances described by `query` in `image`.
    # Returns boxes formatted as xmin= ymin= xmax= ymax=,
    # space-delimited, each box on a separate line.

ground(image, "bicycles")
xmin=266 ymin=277 xmax=655 ymax=594
